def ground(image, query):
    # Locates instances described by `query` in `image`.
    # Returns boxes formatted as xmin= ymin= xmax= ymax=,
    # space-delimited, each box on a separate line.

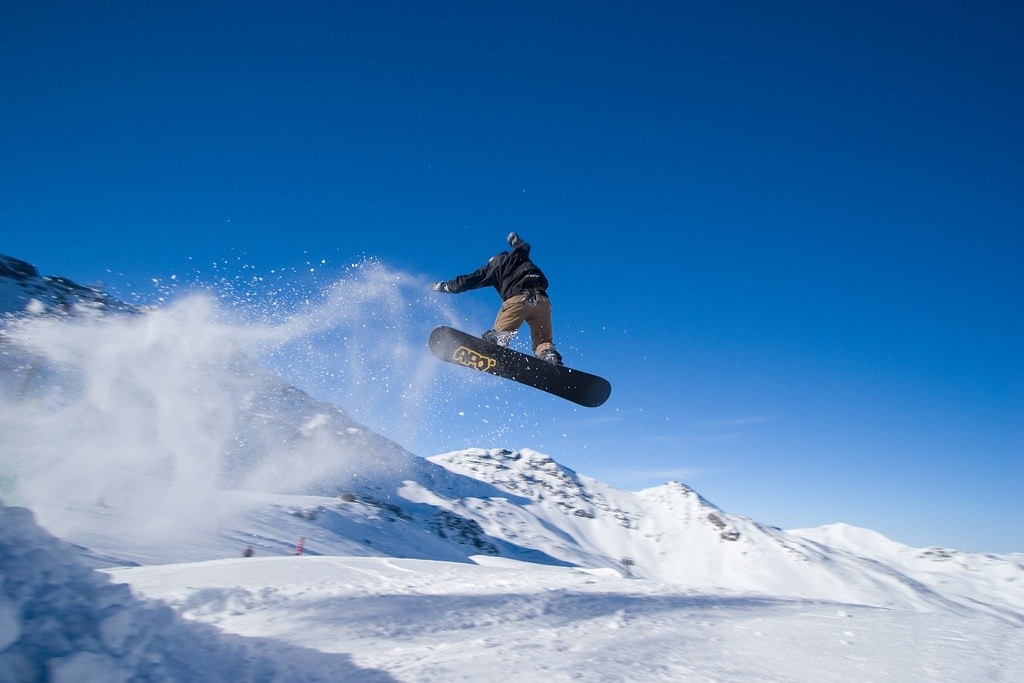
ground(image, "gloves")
xmin=432 ymin=281 xmax=448 ymax=293
xmin=506 ymin=232 xmax=518 ymax=245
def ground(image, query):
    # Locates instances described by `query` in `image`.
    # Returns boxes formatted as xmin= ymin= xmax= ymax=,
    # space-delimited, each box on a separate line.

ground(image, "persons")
xmin=431 ymin=231 xmax=564 ymax=367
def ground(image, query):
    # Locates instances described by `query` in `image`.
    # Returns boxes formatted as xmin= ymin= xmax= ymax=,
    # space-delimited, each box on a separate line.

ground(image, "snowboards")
xmin=426 ymin=325 xmax=613 ymax=410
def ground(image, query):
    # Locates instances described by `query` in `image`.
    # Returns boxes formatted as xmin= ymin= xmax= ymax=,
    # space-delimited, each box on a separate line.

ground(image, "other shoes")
xmin=482 ymin=331 xmax=509 ymax=347
xmin=540 ymin=351 xmax=562 ymax=365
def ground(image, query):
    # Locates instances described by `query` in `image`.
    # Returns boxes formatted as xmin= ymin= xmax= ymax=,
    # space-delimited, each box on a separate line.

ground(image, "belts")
xmin=513 ymin=289 xmax=546 ymax=297
